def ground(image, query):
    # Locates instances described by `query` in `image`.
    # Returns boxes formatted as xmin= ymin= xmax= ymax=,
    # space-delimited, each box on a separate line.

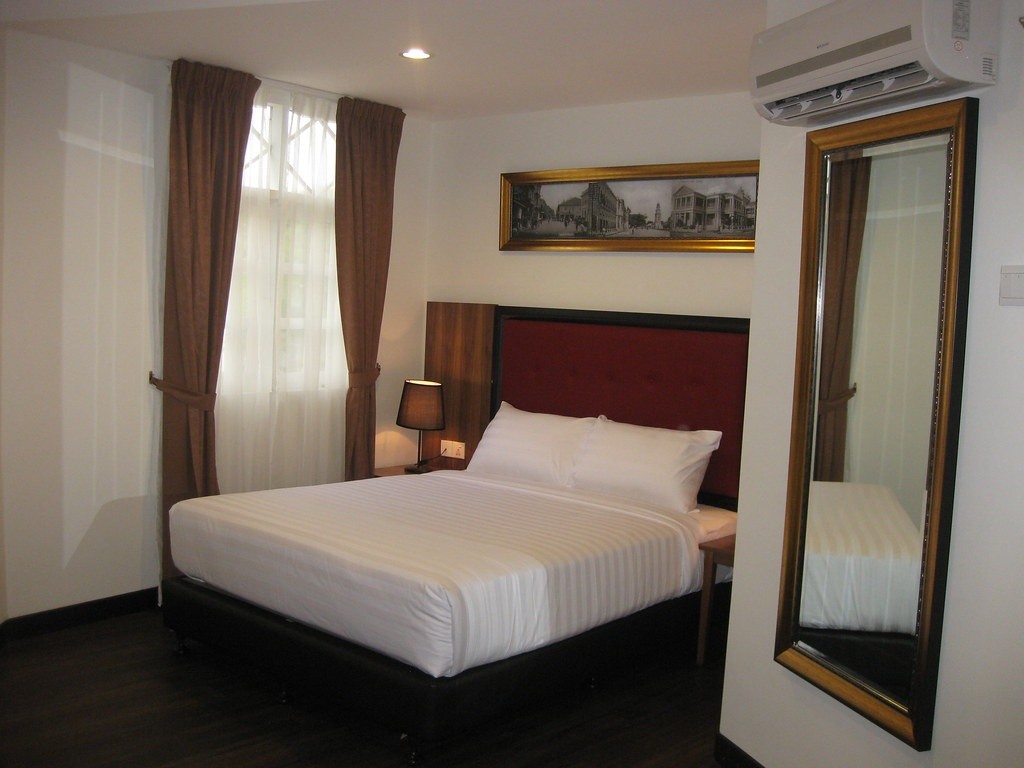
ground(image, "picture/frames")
xmin=499 ymin=160 xmax=760 ymax=253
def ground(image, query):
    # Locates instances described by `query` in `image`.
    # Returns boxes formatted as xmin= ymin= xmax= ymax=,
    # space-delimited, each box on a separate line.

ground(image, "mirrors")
xmin=773 ymin=96 xmax=979 ymax=751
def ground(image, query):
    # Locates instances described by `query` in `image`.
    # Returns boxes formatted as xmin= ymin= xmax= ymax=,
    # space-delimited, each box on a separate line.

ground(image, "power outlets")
xmin=452 ymin=441 xmax=465 ymax=460
xmin=441 ymin=440 xmax=453 ymax=457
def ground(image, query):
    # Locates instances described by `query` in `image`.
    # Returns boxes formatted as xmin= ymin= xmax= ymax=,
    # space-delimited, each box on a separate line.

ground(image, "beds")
xmin=798 ymin=481 xmax=923 ymax=643
xmin=162 ymin=305 xmax=752 ymax=768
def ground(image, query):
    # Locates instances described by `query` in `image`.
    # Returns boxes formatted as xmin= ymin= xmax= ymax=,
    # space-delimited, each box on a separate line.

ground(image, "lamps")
xmin=396 ymin=380 xmax=445 ymax=474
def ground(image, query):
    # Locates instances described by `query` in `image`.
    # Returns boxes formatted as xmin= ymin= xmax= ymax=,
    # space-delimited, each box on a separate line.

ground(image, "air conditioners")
xmin=749 ymin=0 xmax=1000 ymax=127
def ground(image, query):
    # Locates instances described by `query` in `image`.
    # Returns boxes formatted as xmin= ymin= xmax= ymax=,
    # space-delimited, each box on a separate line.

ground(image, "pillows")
xmin=570 ymin=414 xmax=723 ymax=515
xmin=465 ymin=401 xmax=597 ymax=490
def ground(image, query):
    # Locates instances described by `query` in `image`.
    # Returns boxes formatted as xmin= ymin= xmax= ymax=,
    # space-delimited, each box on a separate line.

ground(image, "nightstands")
xmin=370 ymin=465 xmax=441 ymax=477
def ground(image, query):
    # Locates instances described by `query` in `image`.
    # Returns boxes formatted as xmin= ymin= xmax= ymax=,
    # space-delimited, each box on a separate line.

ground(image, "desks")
xmin=695 ymin=533 xmax=737 ymax=667
